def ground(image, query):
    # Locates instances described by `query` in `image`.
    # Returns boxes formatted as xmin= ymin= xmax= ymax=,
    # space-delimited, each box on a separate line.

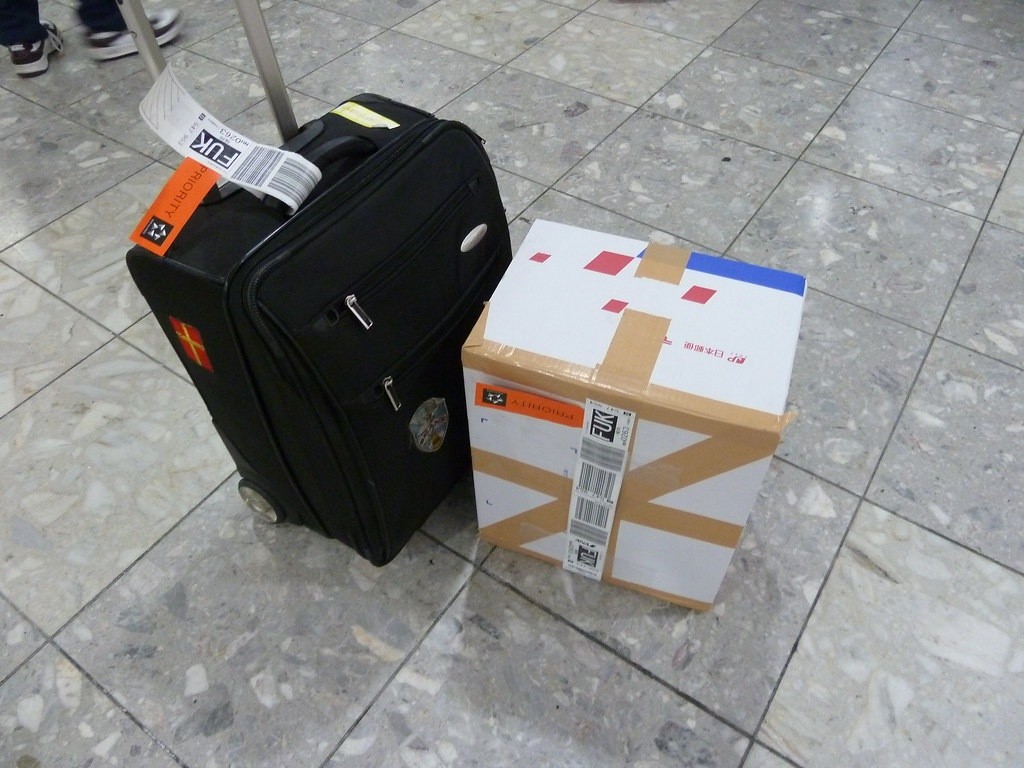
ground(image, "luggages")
xmin=114 ymin=0 xmax=513 ymax=568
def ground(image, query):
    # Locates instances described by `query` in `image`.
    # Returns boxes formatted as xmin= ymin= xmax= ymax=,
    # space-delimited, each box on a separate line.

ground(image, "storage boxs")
xmin=460 ymin=218 xmax=807 ymax=612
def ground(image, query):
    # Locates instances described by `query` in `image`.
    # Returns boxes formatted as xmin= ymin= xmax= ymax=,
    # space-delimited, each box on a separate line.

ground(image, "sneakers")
xmin=85 ymin=9 xmax=185 ymax=60
xmin=7 ymin=18 xmax=63 ymax=78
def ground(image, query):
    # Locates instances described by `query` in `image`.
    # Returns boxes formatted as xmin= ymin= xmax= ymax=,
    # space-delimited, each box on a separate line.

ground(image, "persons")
xmin=0 ymin=0 xmax=184 ymax=79
